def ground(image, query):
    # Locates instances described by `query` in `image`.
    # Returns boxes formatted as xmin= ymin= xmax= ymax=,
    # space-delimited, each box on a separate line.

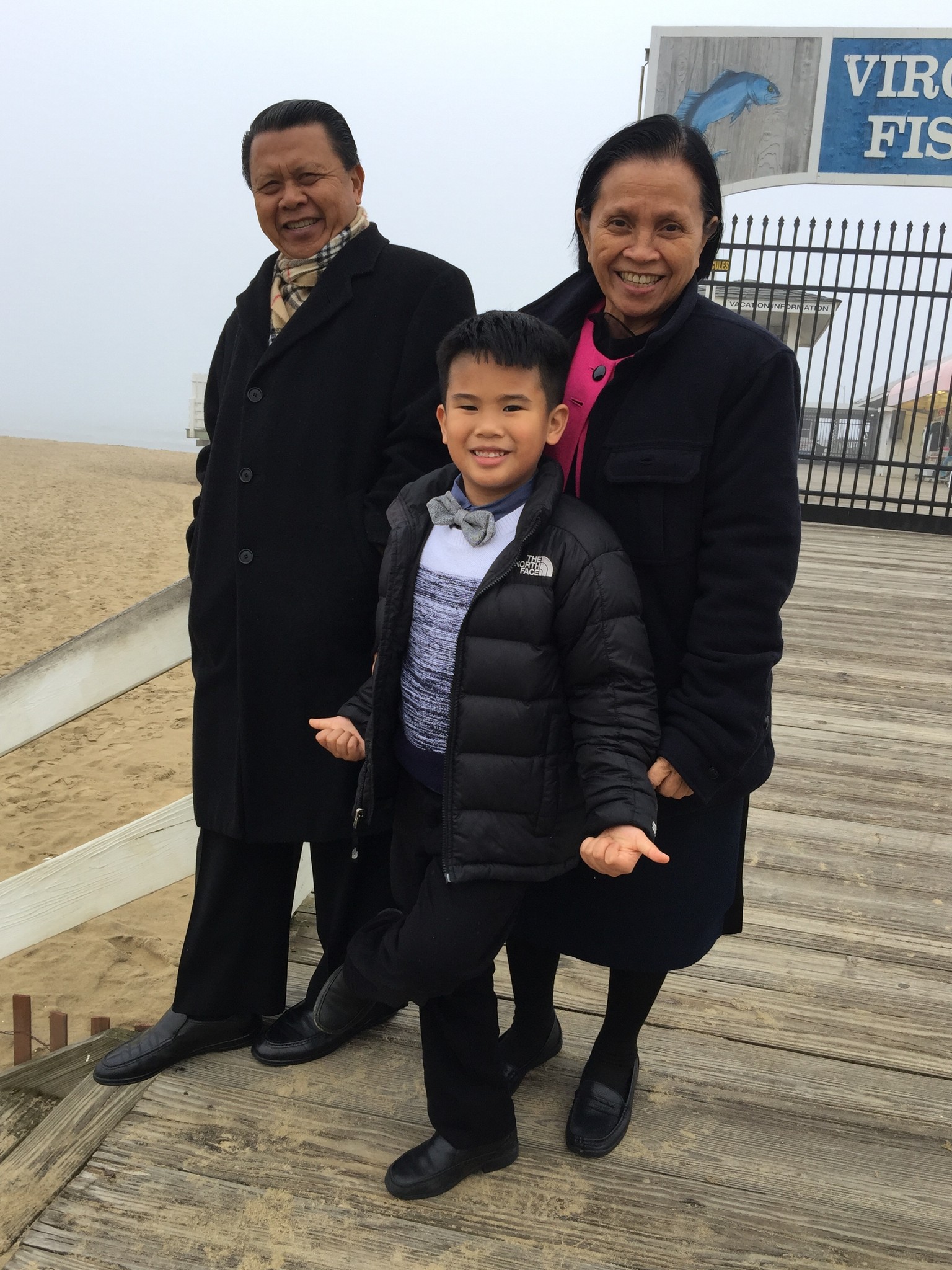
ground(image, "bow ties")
xmin=426 ymin=492 xmax=495 ymax=548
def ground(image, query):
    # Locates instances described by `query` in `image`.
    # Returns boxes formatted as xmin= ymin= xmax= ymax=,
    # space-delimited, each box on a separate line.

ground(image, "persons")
xmin=309 ymin=311 xmax=671 ymax=1202
xmin=91 ymin=100 xmax=478 ymax=1085
xmin=499 ymin=114 xmax=801 ymax=1158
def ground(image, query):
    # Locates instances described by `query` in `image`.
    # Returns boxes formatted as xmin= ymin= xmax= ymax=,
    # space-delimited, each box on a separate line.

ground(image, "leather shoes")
xmin=94 ymin=1006 xmax=255 ymax=1086
xmin=386 ymin=1123 xmax=519 ymax=1200
xmin=312 ymin=964 xmax=374 ymax=1035
xmin=566 ymin=1051 xmax=640 ymax=1156
xmin=251 ymin=996 xmax=398 ymax=1067
xmin=497 ymin=1009 xmax=563 ymax=1098
